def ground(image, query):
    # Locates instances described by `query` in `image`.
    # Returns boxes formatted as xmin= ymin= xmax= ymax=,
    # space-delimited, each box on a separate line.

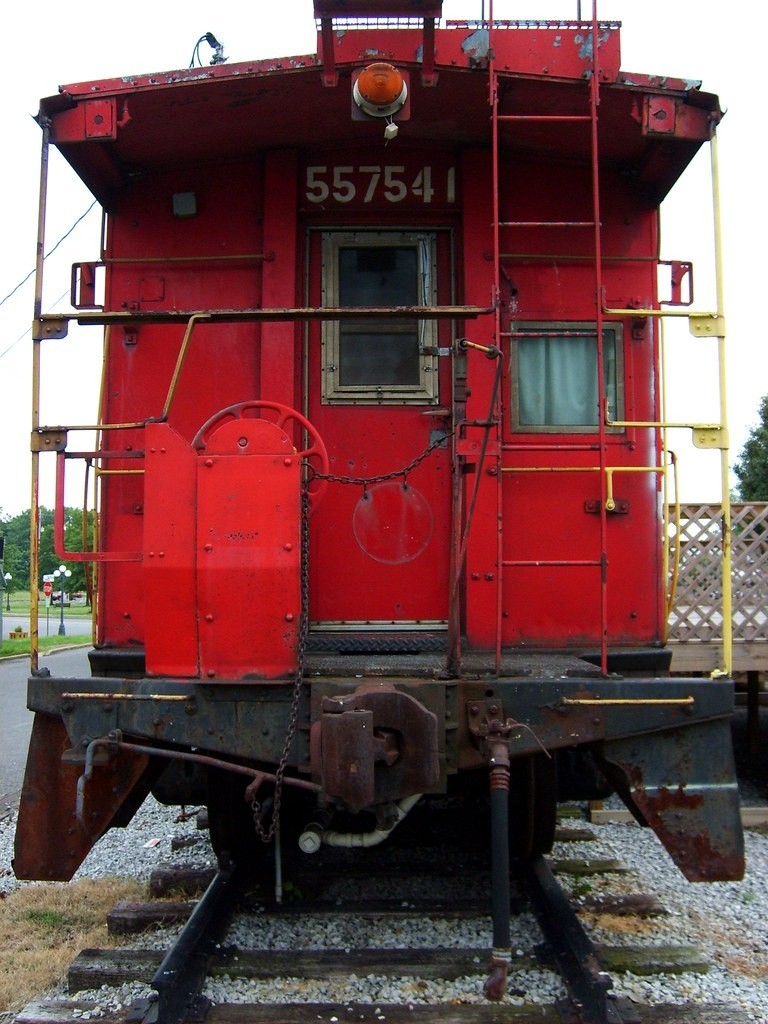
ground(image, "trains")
xmin=12 ymin=0 xmax=744 ymax=998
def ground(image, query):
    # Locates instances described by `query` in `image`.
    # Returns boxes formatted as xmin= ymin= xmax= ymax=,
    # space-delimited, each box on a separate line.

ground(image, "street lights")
xmin=4 ymin=572 xmax=12 ymax=611
xmin=53 ymin=565 xmax=72 ymax=636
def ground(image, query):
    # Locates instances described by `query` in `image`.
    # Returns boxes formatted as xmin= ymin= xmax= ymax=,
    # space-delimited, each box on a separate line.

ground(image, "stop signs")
xmin=43 ymin=582 xmax=53 ymax=596
